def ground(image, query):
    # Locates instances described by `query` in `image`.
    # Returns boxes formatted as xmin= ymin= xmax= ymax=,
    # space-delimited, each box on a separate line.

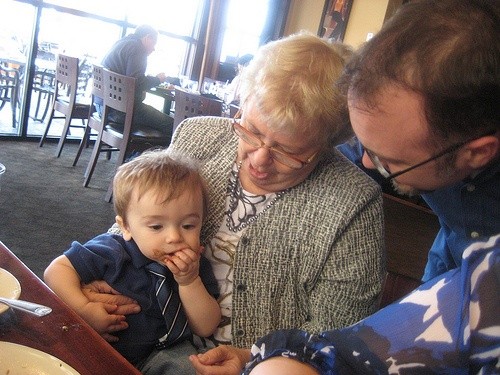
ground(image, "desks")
xmin=0 ymin=241 xmax=144 ymax=375
xmin=146 ymin=89 xmax=223 ymax=117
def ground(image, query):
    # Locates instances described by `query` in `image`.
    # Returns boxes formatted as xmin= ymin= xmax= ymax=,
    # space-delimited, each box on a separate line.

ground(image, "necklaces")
xmin=228 ymin=161 xmax=289 ymax=232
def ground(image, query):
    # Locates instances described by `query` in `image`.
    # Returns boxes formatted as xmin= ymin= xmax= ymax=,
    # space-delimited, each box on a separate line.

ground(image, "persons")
xmin=235 ymin=0 xmax=500 ymax=375
xmin=234 ymin=53 xmax=253 ymax=74
xmin=81 ymin=33 xmax=387 ymax=375
xmin=44 ymin=148 xmax=220 ymax=366
xmin=92 ymin=24 xmax=174 ymax=132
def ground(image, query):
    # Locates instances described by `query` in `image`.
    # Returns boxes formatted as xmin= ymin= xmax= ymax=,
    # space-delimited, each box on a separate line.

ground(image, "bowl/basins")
xmin=0 ymin=267 xmax=22 ymax=313
xmin=0 ymin=341 xmax=81 ymax=375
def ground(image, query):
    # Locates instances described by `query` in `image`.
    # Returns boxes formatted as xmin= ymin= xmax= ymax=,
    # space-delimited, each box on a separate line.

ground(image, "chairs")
xmin=0 ymin=51 xmax=242 ymax=203
xmin=375 ymin=189 xmax=441 ymax=314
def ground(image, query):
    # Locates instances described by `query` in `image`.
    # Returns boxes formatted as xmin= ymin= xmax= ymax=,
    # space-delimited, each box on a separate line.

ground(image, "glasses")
xmin=231 ymin=105 xmax=330 ymax=170
xmin=352 ymin=132 xmax=495 ymax=183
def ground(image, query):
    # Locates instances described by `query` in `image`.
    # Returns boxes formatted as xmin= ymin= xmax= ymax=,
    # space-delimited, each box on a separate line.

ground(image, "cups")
xmin=179 ymin=75 xmax=230 ymax=100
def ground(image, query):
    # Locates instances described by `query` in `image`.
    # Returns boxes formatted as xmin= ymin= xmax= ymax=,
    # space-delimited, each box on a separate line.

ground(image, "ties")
xmin=144 ymin=263 xmax=192 ymax=350
